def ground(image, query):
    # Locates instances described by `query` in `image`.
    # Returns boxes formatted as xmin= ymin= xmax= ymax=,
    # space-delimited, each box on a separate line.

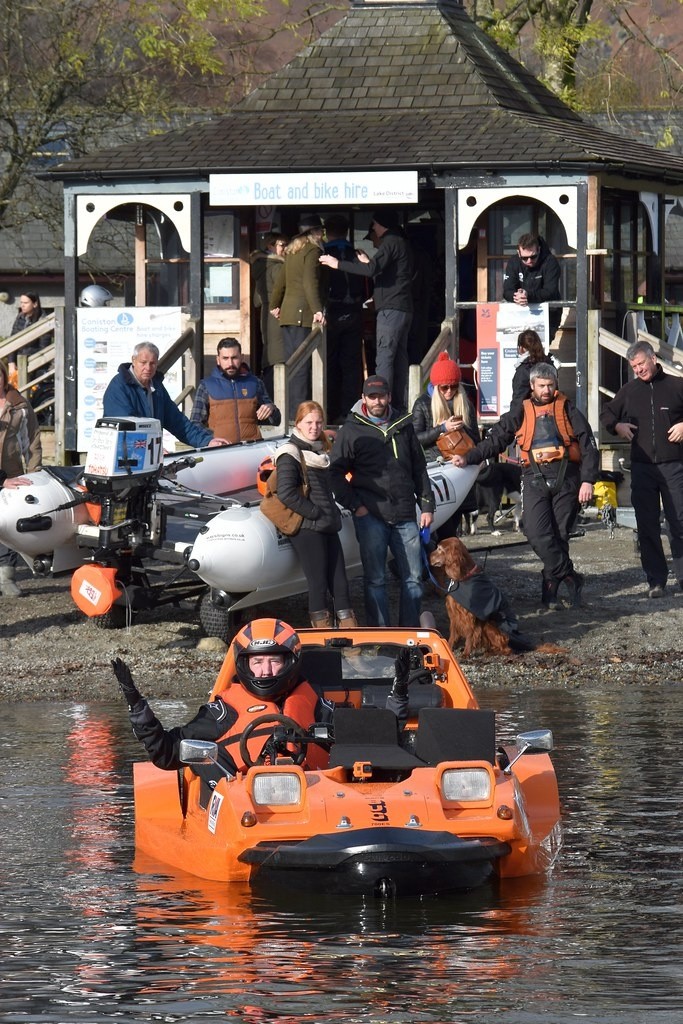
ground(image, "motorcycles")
xmin=133 ymin=628 xmax=563 ymax=895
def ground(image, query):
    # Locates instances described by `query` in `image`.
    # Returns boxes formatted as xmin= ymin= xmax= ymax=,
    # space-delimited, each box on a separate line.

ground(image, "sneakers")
xmin=542 ymin=586 xmax=565 ymax=610
xmin=648 ymin=582 xmax=663 ymax=598
xmin=564 ymin=570 xmax=585 ymax=608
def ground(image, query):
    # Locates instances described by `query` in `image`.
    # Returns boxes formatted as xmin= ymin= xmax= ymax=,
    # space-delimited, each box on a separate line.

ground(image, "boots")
xmin=0 ymin=566 xmax=21 ymax=598
xmin=337 ymin=609 xmax=357 ymax=628
xmin=309 ymin=608 xmax=333 ymax=628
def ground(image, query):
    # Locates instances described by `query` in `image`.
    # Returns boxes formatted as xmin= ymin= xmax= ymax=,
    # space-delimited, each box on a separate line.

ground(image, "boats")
xmin=1 ymin=417 xmax=482 ymax=615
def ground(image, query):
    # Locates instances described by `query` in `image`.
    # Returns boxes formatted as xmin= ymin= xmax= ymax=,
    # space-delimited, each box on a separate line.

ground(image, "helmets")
xmin=256 ymin=456 xmax=277 ymax=497
xmin=233 ymin=618 xmax=303 ymax=702
xmin=80 ymin=286 xmax=113 ymax=308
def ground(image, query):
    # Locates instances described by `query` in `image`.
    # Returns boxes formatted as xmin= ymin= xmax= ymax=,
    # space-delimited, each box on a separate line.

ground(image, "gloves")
xmin=111 ymin=657 xmax=140 ymax=706
xmin=392 ymin=647 xmax=411 ymax=702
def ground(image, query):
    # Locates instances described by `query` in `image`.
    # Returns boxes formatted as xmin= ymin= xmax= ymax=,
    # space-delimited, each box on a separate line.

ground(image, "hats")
xmin=292 ymin=214 xmax=325 ymax=239
xmin=363 ymin=222 xmax=375 ymax=240
xmin=430 ymin=352 xmax=461 ymax=385
xmin=363 ymin=375 xmax=390 ymax=395
xmin=373 ymin=207 xmax=400 ymax=229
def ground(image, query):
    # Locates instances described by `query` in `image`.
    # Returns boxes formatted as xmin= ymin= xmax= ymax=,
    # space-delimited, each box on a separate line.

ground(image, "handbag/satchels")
xmin=436 ymin=428 xmax=476 ymax=461
xmin=259 ymin=442 xmax=309 ymax=535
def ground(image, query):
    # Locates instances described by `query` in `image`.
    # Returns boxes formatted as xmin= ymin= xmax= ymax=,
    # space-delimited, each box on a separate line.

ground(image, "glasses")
xmin=276 ymin=242 xmax=286 ymax=247
xmin=438 ymin=384 xmax=458 ymax=391
xmin=519 ymin=250 xmax=538 ymax=260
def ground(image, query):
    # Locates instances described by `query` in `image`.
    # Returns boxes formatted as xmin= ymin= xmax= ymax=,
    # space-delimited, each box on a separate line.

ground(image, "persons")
xmin=600 ymin=341 xmax=683 ymax=598
xmin=510 ymin=329 xmax=557 ymax=413
xmin=453 ymin=361 xmax=601 ymax=610
xmin=503 ymin=232 xmax=564 ymax=345
xmin=102 ymin=343 xmax=232 ymax=449
xmin=254 ymin=213 xmax=326 ymax=423
xmin=273 ymin=400 xmax=358 ymax=628
xmin=316 ymin=213 xmax=366 ymax=424
xmin=7 ymin=289 xmax=52 ymax=425
xmin=110 ymin=618 xmax=337 ymax=790
xmin=326 ymin=374 xmax=436 ymax=628
xmin=0 ymin=359 xmax=43 ymax=490
xmin=412 ymin=351 xmax=479 ymax=544
xmin=318 ymin=205 xmax=421 ymax=412
xmin=81 ymin=285 xmax=114 ymax=307
xmin=190 ymin=337 xmax=281 ymax=445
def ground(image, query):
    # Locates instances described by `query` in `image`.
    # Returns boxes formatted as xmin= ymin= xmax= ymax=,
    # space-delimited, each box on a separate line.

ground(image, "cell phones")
xmin=453 ymin=415 xmax=463 ymax=422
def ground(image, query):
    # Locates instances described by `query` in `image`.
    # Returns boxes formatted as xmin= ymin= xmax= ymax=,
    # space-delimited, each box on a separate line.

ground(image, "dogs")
xmin=469 ymin=462 xmax=523 ymax=536
xmin=428 ymin=537 xmax=573 ymax=660
